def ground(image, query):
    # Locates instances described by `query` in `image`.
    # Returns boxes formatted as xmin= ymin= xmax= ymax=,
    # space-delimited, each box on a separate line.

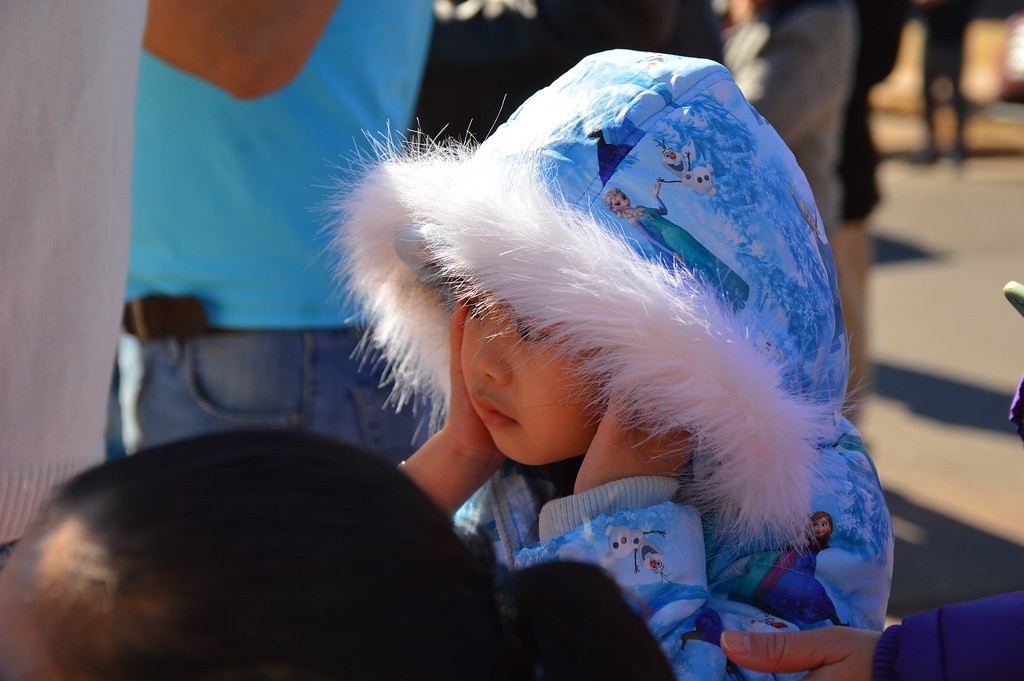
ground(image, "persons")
xmin=0 ymin=0 xmax=339 ymax=547
xmin=422 ymin=0 xmax=902 ymax=409
xmin=0 ymin=429 xmax=675 ymax=681
xmin=115 ymin=0 xmax=435 ymax=456
xmin=335 ymin=46 xmax=896 ymax=681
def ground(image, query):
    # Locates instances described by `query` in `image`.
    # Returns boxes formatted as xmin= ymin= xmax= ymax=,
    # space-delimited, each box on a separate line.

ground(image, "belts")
xmin=118 ymin=293 xmax=238 ymax=336
